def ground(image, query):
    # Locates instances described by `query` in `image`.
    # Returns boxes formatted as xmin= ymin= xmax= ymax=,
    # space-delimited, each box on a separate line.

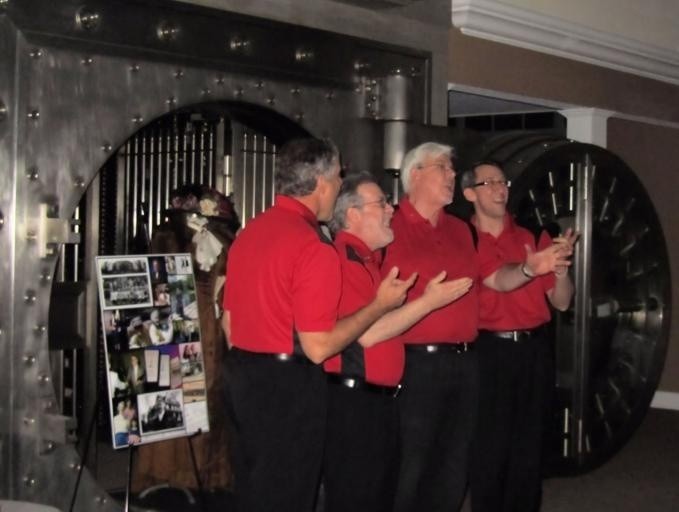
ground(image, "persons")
xmin=455 ymin=159 xmax=580 ymax=510
xmin=218 ymin=133 xmax=420 ymax=509
xmin=128 ymin=180 xmax=241 ymax=494
xmin=322 ymin=168 xmax=476 ymax=512
xmin=386 ymin=135 xmax=575 ymax=511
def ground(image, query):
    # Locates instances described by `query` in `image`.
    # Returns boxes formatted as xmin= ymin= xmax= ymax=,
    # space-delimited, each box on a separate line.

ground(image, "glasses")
xmin=473 ymin=179 xmax=512 ymax=187
xmin=364 ymin=194 xmax=390 ymax=208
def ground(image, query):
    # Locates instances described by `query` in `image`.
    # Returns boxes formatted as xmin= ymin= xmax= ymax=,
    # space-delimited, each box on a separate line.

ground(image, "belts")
xmin=409 ymin=342 xmax=468 ymax=353
xmin=231 ymin=347 xmax=313 ymax=363
xmin=327 ymin=373 xmax=408 ymax=398
xmin=495 ymin=330 xmax=533 ymax=343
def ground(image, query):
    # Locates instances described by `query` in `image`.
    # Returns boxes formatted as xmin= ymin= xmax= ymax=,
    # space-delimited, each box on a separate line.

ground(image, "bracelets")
xmin=518 ymin=254 xmax=536 ymax=281
xmin=551 ymin=265 xmax=568 ymax=281
